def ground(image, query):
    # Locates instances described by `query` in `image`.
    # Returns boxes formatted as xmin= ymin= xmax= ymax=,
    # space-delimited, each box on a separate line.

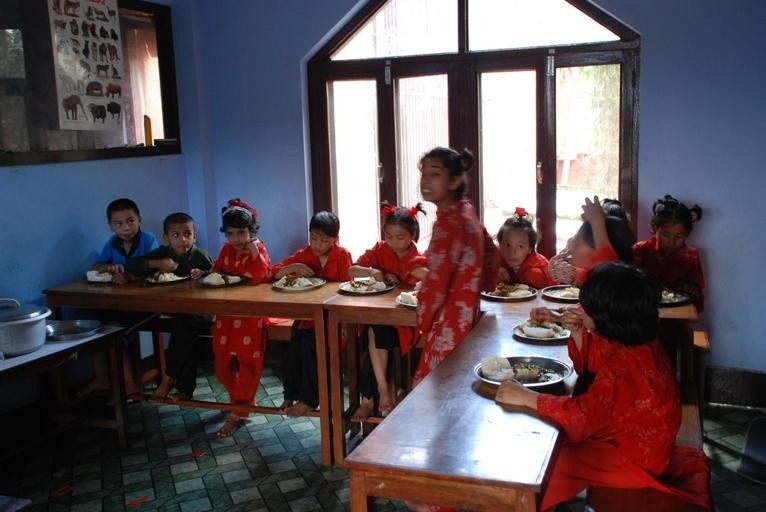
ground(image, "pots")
xmin=0 ymin=297 xmax=51 ymax=358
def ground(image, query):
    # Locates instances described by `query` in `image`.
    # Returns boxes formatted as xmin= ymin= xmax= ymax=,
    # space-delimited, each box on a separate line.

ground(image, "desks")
xmin=0 ymin=320 xmax=134 ymax=453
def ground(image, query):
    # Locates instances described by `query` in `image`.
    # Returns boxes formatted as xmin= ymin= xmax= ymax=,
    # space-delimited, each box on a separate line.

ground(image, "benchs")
xmin=676 ymin=402 xmax=701 ymax=453
xmin=155 ymin=312 xmax=293 ymax=340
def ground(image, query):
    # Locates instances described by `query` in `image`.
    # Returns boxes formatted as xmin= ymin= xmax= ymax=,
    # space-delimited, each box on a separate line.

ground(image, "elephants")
xmin=63 ymin=95 xmax=88 ymax=121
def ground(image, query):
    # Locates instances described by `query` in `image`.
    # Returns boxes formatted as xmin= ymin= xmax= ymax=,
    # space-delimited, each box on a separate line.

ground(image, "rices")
xmin=482 ymin=354 xmax=512 ymax=382
xmin=562 ymin=286 xmax=581 ymax=298
xmin=353 ymin=276 xmax=384 ymax=291
xmin=204 ymin=273 xmax=224 ymax=286
xmin=507 ymin=283 xmax=531 ymax=297
xmin=524 ymin=324 xmax=553 ymax=338
xmin=400 ymin=291 xmax=419 ymax=306
xmin=158 ymin=272 xmax=180 ymax=282
xmin=277 ymin=276 xmax=310 ymax=288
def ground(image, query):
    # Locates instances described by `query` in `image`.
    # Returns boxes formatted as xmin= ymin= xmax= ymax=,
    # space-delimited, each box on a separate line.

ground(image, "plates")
xmin=510 ymin=322 xmax=572 ymax=341
xmin=197 ymin=271 xmax=253 ymax=289
xmin=394 ymin=289 xmax=419 ymax=308
xmin=473 ymin=354 xmax=574 ymax=387
xmin=47 ymin=317 xmax=103 ymax=342
xmin=658 ymin=296 xmax=695 ymax=308
xmin=338 ymin=279 xmax=398 ymax=294
xmin=540 ymin=283 xmax=581 ymax=304
xmin=141 ymin=271 xmax=191 ymax=285
xmin=271 ymin=276 xmax=327 ymax=294
xmin=84 ymin=277 xmax=114 ymax=286
xmin=480 ymin=287 xmax=539 ymax=303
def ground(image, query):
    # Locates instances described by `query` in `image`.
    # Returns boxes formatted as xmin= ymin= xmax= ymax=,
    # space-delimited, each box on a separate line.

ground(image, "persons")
xmin=212 ymin=198 xmax=270 ymax=438
xmin=637 ymin=192 xmax=707 ymax=312
xmin=92 ymin=198 xmax=160 ymax=401
xmin=549 ymin=195 xmax=635 ymax=286
xmin=412 ymin=146 xmax=503 ymax=388
xmin=351 ymin=201 xmax=426 ymax=424
xmin=125 ymin=212 xmax=211 ymax=401
xmin=488 ymin=207 xmax=559 ymax=293
xmin=271 ymin=212 xmax=354 ymax=418
xmin=496 ymin=262 xmax=681 ymax=512
